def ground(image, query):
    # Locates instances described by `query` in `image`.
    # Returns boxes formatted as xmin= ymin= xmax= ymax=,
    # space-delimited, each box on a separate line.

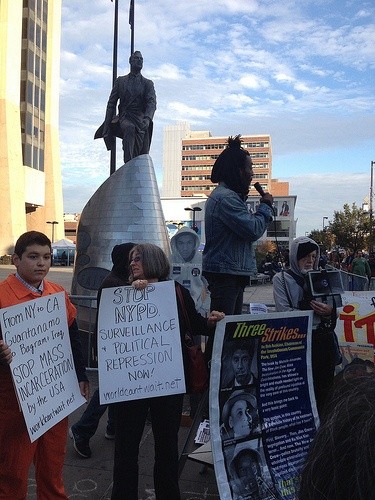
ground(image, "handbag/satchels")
xmin=176 ymin=285 xmax=210 ymax=394
xmin=332 ymin=331 xmax=342 ymax=366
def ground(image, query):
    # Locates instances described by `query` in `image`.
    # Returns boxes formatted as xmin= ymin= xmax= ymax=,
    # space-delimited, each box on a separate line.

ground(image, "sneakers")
xmin=105 ymin=426 xmax=115 ymax=439
xmin=69 ymin=425 xmax=91 ymax=458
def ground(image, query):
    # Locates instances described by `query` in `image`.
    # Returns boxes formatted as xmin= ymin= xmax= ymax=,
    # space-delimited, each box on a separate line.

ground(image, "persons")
xmin=274 ymin=236 xmax=342 ymax=426
xmin=62 ymin=251 xmax=67 ymax=266
xmin=258 ymin=248 xmax=290 ymax=284
xmin=166 ymin=226 xmax=211 ymax=316
xmin=319 ymin=245 xmax=375 ymax=291
xmin=93 ymin=51 xmax=157 ymax=164
xmin=66 ymin=242 xmax=141 ymax=459
xmin=227 ymin=443 xmax=265 ymax=500
xmin=246 ymin=201 xmax=289 ymax=216
xmin=223 ymin=340 xmax=260 ymax=387
xmin=0 ymin=231 xmax=89 ymax=500
xmin=220 ymin=389 xmax=261 ymax=440
xmin=202 ymin=134 xmax=273 ymax=357
xmin=293 ymin=387 xmax=375 ymax=500
xmin=106 ymin=244 xmax=225 ymax=500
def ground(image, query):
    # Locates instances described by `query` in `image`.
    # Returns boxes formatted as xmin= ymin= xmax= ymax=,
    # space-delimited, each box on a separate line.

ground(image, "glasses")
xmin=129 ymin=256 xmax=141 ymax=265
xmin=304 ymin=254 xmax=316 ymax=260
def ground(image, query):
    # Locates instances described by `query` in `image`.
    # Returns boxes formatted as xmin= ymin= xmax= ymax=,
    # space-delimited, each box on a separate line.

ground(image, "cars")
xmin=327 ymin=244 xmax=363 ymax=258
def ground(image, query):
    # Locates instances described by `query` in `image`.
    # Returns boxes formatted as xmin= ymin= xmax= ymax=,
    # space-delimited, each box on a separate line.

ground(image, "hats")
xmin=222 ymin=391 xmax=257 ymax=429
xmin=339 ymin=249 xmax=345 ymax=254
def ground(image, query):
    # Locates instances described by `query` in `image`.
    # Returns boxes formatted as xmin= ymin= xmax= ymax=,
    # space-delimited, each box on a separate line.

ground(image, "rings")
xmin=220 ymin=311 xmax=224 ymax=316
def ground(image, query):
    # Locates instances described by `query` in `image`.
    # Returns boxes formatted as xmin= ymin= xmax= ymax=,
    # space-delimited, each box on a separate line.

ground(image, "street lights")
xmin=322 ymin=216 xmax=328 ymax=231
xmin=184 ymin=206 xmax=202 ymax=230
xmin=370 ymin=160 xmax=375 ymax=234
xmin=46 ymin=221 xmax=59 ymax=243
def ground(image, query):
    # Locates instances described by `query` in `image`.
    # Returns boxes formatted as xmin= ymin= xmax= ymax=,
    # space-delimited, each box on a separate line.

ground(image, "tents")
xmin=49 ymin=237 xmax=75 ymax=267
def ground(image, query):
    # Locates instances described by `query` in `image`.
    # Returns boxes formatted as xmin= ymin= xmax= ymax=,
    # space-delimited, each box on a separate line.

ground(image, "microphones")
xmin=254 ymin=182 xmax=274 ymax=210
